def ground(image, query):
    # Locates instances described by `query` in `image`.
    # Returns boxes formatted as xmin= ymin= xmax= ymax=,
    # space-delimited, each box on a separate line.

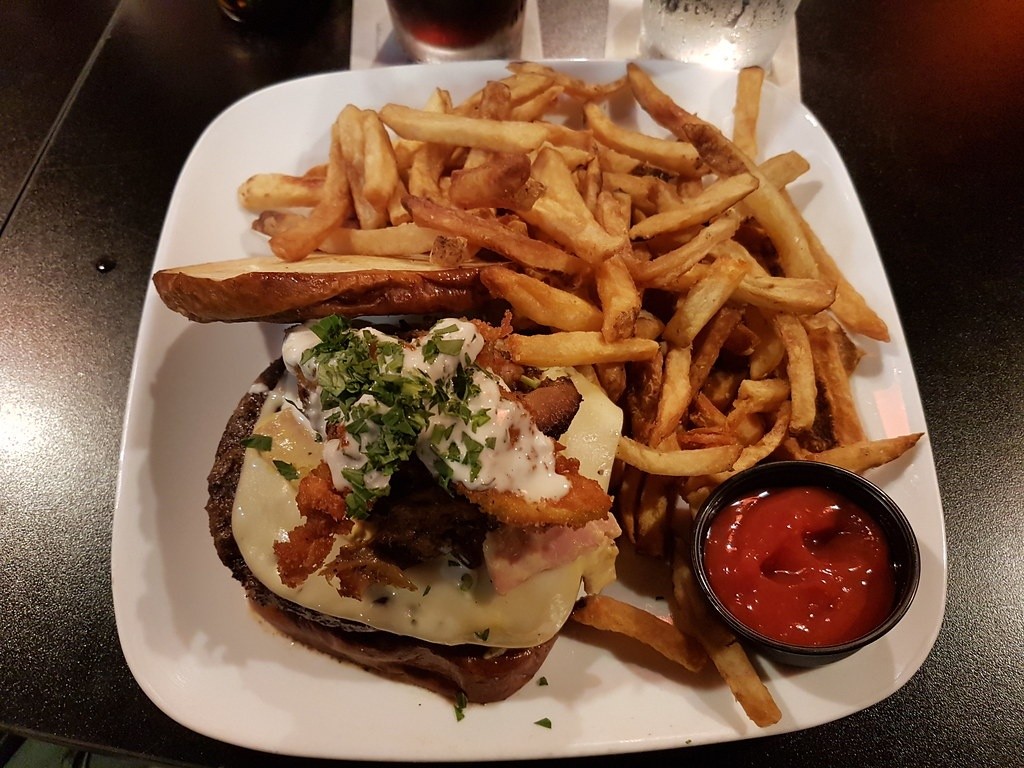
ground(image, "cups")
xmin=638 ymin=0 xmax=800 ymax=69
xmin=387 ymin=0 xmax=529 ymax=63
xmin=691 ymin=460 xmax=921 ymax=666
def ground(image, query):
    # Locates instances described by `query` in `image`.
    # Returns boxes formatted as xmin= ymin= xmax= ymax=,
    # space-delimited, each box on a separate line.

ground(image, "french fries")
xmin=236 ymin=60 xmax=928 ymax=727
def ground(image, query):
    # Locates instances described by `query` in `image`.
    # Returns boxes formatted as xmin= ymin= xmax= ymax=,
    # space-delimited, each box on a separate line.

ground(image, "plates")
xmin=110 ymin=57 xmax=948 ymax=759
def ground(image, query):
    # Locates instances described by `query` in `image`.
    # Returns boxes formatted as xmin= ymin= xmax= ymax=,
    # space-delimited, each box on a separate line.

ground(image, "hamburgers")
xmin=148 ymin=251 xmax=625 ymax=711
